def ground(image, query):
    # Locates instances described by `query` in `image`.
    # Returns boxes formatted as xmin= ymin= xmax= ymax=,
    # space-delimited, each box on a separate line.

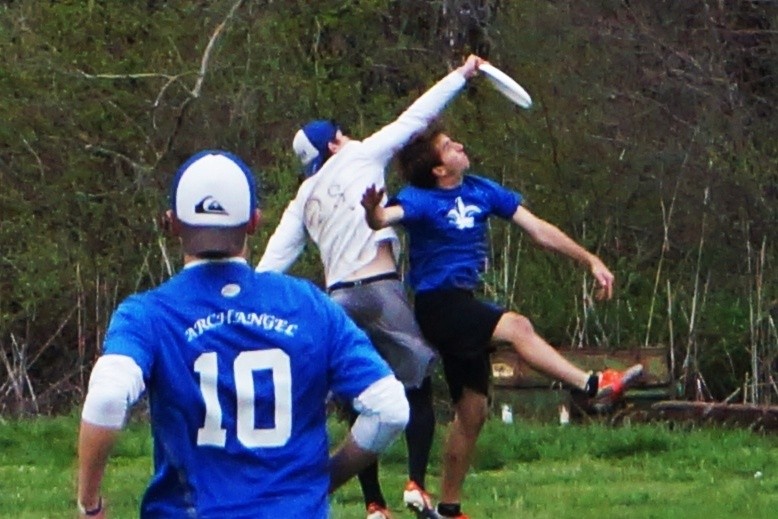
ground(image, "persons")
xmin=78 ymin=149 xmax=409 ymax=519
xmin=360 ymin=125 xmax=642 ymax=519
xmin=254 ymin=54 xmax=483 ymax=519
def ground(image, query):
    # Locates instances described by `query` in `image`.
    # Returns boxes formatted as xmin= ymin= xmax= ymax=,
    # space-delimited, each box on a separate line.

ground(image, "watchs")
xmin=79 ymin=497 xmax=102 ymax=516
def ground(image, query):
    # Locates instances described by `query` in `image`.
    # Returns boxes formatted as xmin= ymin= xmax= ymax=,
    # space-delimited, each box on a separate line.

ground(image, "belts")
xmin=327 ymin=272 xmax=399 ymax=295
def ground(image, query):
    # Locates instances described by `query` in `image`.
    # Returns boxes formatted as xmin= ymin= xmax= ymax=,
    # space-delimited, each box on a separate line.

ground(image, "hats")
xmin=172 ymin=150 xmax=255 ymax=228
xmin=292 ymin=120 xmax=337 ymax=176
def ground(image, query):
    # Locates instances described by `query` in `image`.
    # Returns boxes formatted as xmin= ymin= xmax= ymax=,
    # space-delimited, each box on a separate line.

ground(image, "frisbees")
xmin=478 ymin=62 xmax=533 ymax=109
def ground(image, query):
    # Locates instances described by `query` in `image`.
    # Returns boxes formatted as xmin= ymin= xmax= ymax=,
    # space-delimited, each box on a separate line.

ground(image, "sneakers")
xmin=588 ymin=364 xmax=644 ymax=416
xmin=433 ymin=503 xmax=468 ymax=519
xmin=367 ymin=502 xmax=391 ymax=519
xmin=404 ymin=481 xmax=433 ymax=519
